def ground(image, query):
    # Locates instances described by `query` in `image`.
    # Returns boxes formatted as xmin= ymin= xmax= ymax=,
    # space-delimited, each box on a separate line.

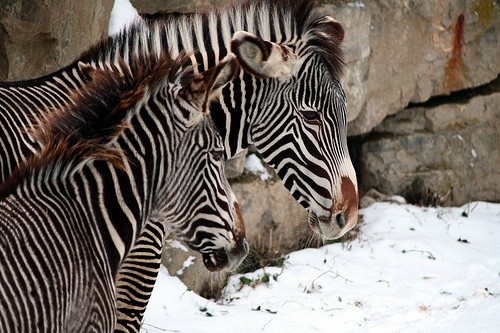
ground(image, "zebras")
xmin=0 ymin=0 xmax=361 ymax=250
xmin=0 ymin=46 xmax=252 ymax=333
xmin=114 ymin=145 xmax=249 ymax=333
